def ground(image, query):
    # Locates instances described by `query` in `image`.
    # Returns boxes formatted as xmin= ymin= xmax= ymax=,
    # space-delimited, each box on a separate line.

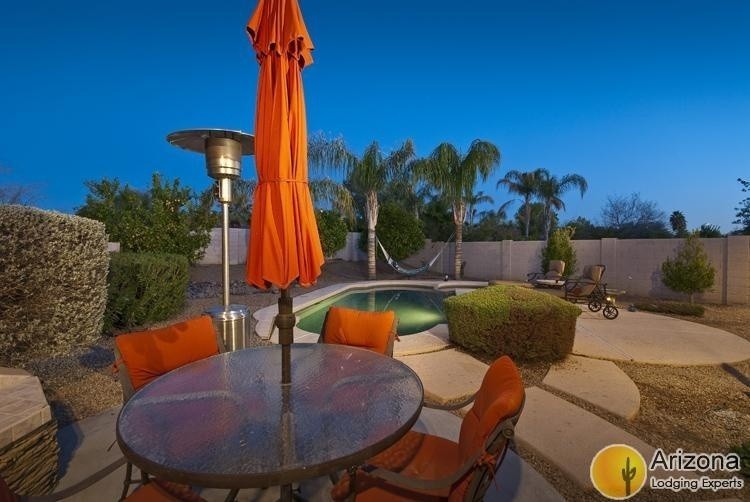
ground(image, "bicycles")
xmin=587 ymin=282 xmax=619 ymax=320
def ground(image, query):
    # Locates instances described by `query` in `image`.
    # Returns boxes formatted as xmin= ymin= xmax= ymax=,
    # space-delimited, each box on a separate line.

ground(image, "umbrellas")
xmin=243 ymin=1 xmax=326 ymax=391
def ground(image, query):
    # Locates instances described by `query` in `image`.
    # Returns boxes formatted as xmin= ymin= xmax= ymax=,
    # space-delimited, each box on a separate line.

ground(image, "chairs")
xmin=526 ymin=260 xmax=565 ymax=288
xmin=112 ymin=306 xmax=526 ymax=501
xmin=564 ymin=264 xmax=618 ymax=319
